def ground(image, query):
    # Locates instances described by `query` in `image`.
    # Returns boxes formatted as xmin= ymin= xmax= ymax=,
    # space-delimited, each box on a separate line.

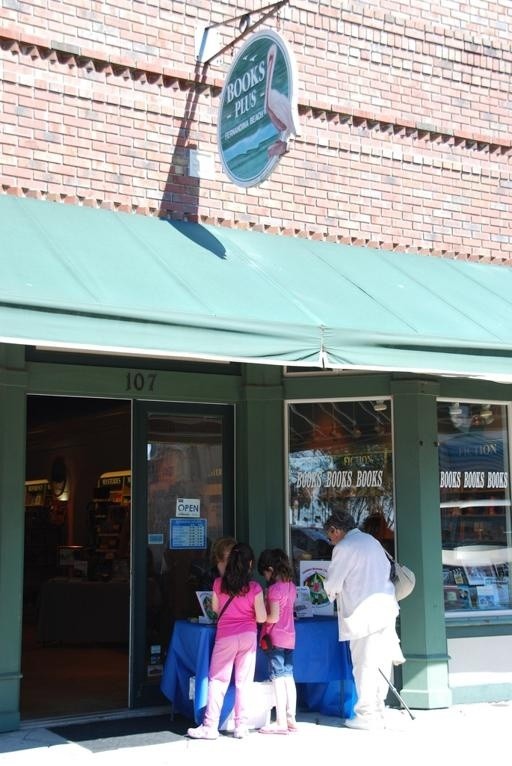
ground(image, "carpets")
xmin=46 ymin=714 xmax=195 ymax=754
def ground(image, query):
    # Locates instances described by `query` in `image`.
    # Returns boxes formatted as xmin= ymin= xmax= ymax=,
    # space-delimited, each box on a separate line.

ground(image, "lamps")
xmin=480 ymin=404 xmax=493 ymax=416
xmin=449 ymin=402 xmax=462 ymax=415
xmin=374 ymin=400 xmax=388 ymax=411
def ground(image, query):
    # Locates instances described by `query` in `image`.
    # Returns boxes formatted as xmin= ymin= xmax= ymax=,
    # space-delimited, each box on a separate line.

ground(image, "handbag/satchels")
xmin=390 ymin=562 xmax=416 ymax=602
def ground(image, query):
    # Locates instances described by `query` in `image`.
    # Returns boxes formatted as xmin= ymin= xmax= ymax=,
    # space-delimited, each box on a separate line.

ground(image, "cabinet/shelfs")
xmin=86 ymin=470 xmax=131 ymax=581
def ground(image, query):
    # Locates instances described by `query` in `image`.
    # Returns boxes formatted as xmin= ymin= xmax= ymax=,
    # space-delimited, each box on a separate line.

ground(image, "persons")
xmin=322 ymin=510 xmax=400 ymax=733
xmin=192 ymin=536 xmax=236 ymax=619
xmin=187 ymin=541 xmax=267 ymax=739
xmin=258 ymin=547 xmax=299 ymax=734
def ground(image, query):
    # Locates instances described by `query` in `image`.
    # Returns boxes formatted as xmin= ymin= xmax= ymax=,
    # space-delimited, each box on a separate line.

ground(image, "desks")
xmin=35 ymin=576 xmax=130 ymax=647
xmin=158 ymin=616 xmax=358 ymax=731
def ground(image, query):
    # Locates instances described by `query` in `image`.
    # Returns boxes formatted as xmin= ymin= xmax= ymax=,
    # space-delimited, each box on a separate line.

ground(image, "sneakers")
xmin=343 ymin=716 xmax=384 ymax=733
xmin=233 ymin=723 xmax=251 ymax=739
xmin=187 ymin=723 xmax=221 ymax=740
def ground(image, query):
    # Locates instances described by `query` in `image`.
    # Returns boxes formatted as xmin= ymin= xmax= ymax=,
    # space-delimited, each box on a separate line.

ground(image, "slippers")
xmin=258 ymin=725 xmax=298 ymax=734
